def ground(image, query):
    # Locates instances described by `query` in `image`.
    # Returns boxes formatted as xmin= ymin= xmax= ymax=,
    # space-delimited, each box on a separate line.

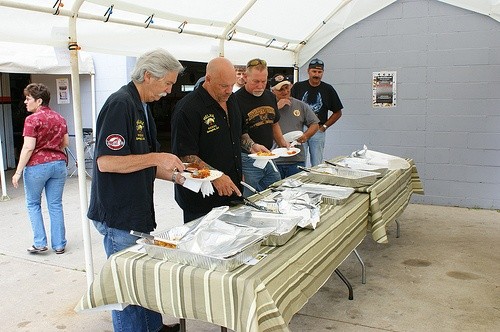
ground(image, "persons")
xmin=233 ymin=66 xmax=246 ymax=93
xmin=290 ymin=58 xmax=344 ymax=167
xmin=12 ymin=82 xmax=69 ymax=254
xmin=270 ymin=73 xmax=320 ymax=179
xmin=87 ymin=48 xmax=186 ymax=332
xmin=235 ymin=59 xmax=297 ymax=196
xmin=172 ymin=57 xmax=245 ymax=223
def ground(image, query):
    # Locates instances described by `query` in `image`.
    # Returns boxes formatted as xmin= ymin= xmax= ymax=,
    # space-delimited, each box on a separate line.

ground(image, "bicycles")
xmin=65 ymin=128 xmax=95 ymax=179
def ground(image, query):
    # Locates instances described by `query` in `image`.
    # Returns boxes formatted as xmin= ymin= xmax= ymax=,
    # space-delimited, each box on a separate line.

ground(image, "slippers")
xmin=27 ymin=244 xmax=48 ymax=253
xmin=55 ymin=249 xmax=64 ymax=254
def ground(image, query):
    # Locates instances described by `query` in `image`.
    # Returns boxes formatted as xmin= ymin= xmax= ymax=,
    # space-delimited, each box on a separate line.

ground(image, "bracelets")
xmin=172 ymin=172 xmax=179 ymax=182
xmin=323 ymin=124 xmax=328 ymax=129
xmin=16 ymin=171 xmax=22 ymax=175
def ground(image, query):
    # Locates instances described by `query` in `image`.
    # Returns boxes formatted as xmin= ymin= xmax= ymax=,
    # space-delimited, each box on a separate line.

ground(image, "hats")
xmin=270 ymin=74 xmax=290 ymax=90
xmin=309 ymin=58 xmax=324 ymax=71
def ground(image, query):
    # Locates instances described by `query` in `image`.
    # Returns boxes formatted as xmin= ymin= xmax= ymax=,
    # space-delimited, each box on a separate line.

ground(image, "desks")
xmin=74 ymin=154 xmax=424 ymax=332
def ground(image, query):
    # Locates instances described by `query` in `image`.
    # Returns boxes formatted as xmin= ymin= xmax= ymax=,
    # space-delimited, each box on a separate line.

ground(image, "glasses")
xmin=309 ymin=59 xmax=324 ymax=65
xmin=247 ymin=60 xmax=266 ymax=68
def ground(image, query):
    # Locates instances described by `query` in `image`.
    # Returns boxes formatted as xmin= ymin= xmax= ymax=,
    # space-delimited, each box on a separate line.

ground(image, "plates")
xmin=183 ymin=170 xmax=223 ymax=180
xmin=272 ymin=148 xmax=300 ymax=158
xmin=247 ymin=153 xmax=278 ymax=160
xmin=282 ymin=130 xmax=303 ymax=142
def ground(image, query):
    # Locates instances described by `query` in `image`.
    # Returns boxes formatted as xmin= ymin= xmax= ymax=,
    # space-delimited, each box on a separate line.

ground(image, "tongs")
xmin=230 ymin=194 xmax=266 ymax=210
xmin=297 ymin=165 xmax=332 ymax=174
xmin=178 ymin=169 xmax=199 ymax=175
